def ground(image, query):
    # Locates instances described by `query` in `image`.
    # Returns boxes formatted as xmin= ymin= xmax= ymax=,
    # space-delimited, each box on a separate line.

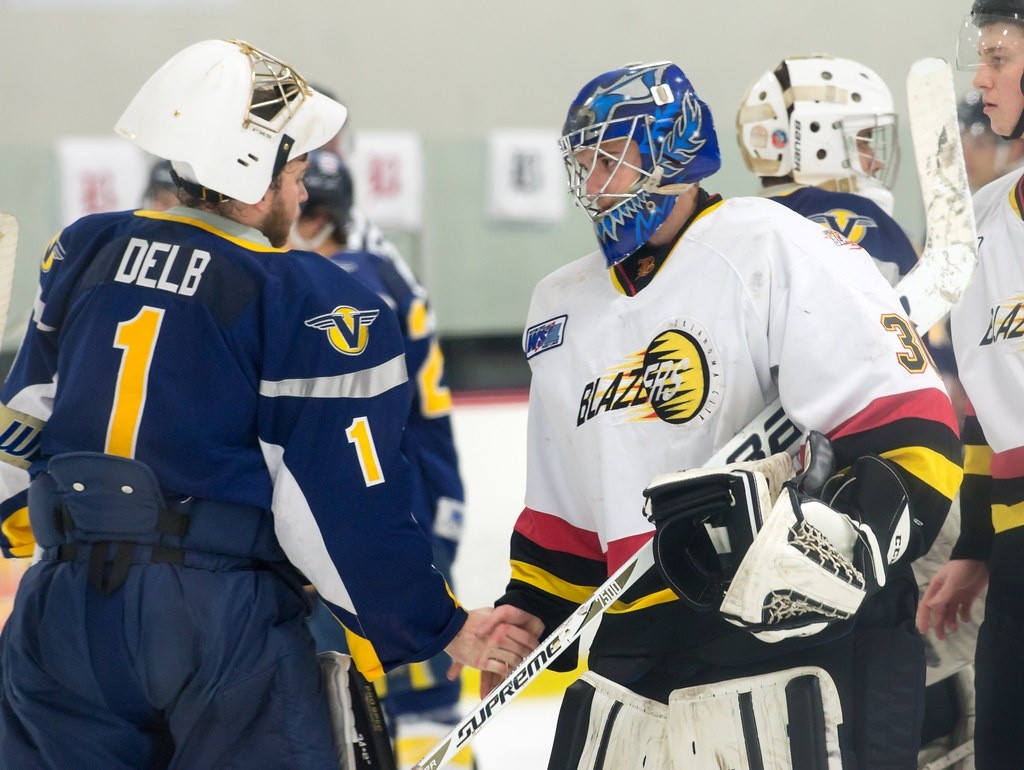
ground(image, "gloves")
xmin=639 ymin=430 xmax=915 ymax=645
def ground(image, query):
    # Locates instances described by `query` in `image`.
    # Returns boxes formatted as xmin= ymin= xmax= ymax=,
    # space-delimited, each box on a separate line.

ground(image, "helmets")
xmin=969 ymin=0 xmax=1023 ymax=25
xmin=555 ymin=59 xmax=722 ymax=272
xmin=110 ymin=38 xmax=350 ymax=206
xmin=734 ymin=54 xmax=904 ymax=217
xmin=302 ymin=148 xmax=354 ymax=218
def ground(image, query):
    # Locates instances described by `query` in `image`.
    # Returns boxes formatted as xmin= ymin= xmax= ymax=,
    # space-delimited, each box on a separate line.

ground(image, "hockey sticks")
xmin=381 ymin=59 xmax=979 ymax=770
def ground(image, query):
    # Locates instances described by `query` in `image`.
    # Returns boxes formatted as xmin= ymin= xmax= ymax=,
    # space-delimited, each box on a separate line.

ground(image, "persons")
xmin=447 ymin=0 xmax=1024 ymax=770
xmin=0 ymin=39 xmax=539 ymax=770
